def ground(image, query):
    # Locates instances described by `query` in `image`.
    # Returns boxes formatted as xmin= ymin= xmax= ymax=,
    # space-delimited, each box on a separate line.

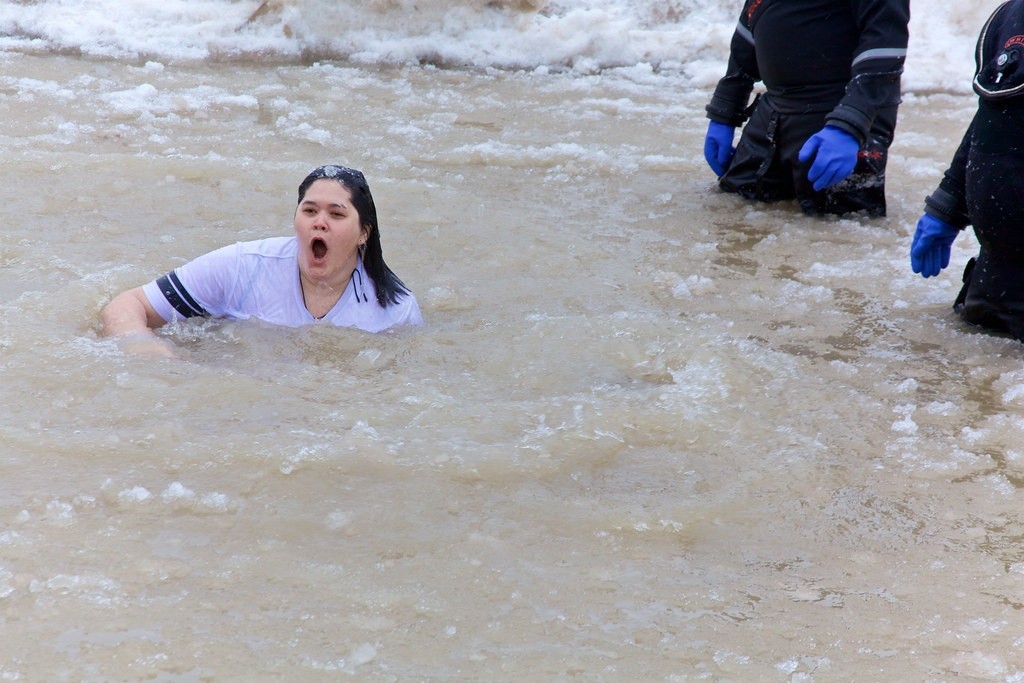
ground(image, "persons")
xmin=100 ymin=166 xmax=426 ymax=364
xmin=703 ymin=0 xmax=911 ymax=221
xmin=907 ymin=0 xmax=1024 ymax=344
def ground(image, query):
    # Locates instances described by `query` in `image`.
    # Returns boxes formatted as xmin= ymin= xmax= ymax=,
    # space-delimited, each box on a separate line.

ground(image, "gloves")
xmin=910 ymin=213 xmax=960 ymax=278
xmin=703 ymin=119 xmax=738 ymax=180
xmin=798 ymin=126 xmax=859 ymax=192
